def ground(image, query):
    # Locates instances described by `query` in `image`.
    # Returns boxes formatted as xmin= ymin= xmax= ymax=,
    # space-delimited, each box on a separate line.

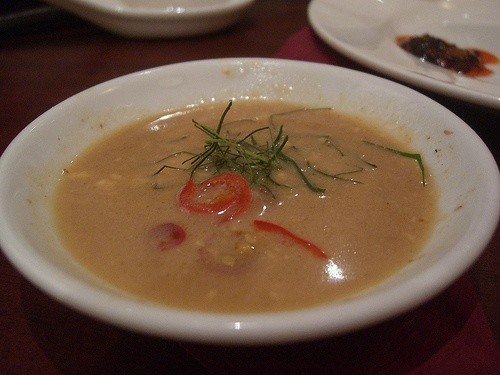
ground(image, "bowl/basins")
xmin=0 ymin=56 xmax=500 ymax=346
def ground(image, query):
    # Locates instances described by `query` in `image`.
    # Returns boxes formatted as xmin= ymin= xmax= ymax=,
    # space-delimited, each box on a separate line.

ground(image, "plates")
xmin=47 ymin=0 xmax=256 ymax=38
xmin=306 ymin=0 xmax=500 ymax=111
